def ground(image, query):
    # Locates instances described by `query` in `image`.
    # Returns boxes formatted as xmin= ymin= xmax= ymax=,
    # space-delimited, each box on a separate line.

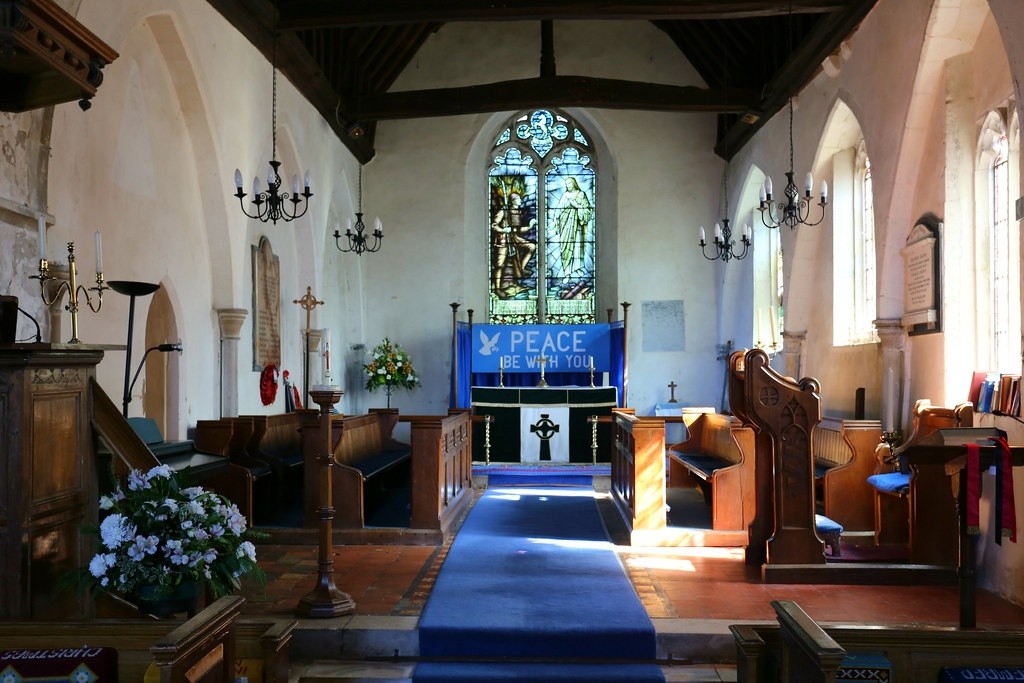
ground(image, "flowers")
xmin=361 ymin=337 xmax=421 ymax=393
xmin=53 ymin=464 xmax=272 ymax=601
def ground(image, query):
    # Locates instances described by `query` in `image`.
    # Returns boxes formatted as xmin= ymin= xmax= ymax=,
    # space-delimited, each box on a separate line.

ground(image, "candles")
xmin=38 ymin=215 xmax=47 ymax=260
xmin=500 ymin=357 xmax=503 ymax=368
xmin=541 ymin=352 xmax=544 ymax=359
xmin=95 ymin=230 xmax=102 ymax=273
xmin=591 ymin=357 xmax=593 ymax=368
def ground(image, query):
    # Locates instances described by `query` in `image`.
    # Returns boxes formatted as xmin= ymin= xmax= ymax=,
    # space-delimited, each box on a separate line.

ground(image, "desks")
xmin=470 ymin=386 xmax=618 ymax=464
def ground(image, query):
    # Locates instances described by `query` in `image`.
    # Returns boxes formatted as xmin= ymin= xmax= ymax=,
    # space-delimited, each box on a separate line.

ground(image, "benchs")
xmin=813 ymin=400 xmax=973 ymax=565
xmin=668 ymin=407 xmax=756 ymax=530
xmin=197 ymin=409 xmax=411 ymax=529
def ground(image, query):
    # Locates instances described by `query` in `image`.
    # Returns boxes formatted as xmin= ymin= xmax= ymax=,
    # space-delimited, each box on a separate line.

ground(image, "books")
xmin=918 ymin=427 xmax=1001 ymax=446
xmin=966 ymin=371 xmax=1022 ymax=418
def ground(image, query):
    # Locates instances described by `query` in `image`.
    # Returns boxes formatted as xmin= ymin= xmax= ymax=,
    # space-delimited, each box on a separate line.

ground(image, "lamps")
xmin=757 ymin=1 xmax=827 ymax=231
xmin=234 ymin=0 xmax=314 ymax=225
xmin=333 ymin=156 xmax=384 ymax=257
xmin=698 ymin=41 xmax=752 ymax=264
